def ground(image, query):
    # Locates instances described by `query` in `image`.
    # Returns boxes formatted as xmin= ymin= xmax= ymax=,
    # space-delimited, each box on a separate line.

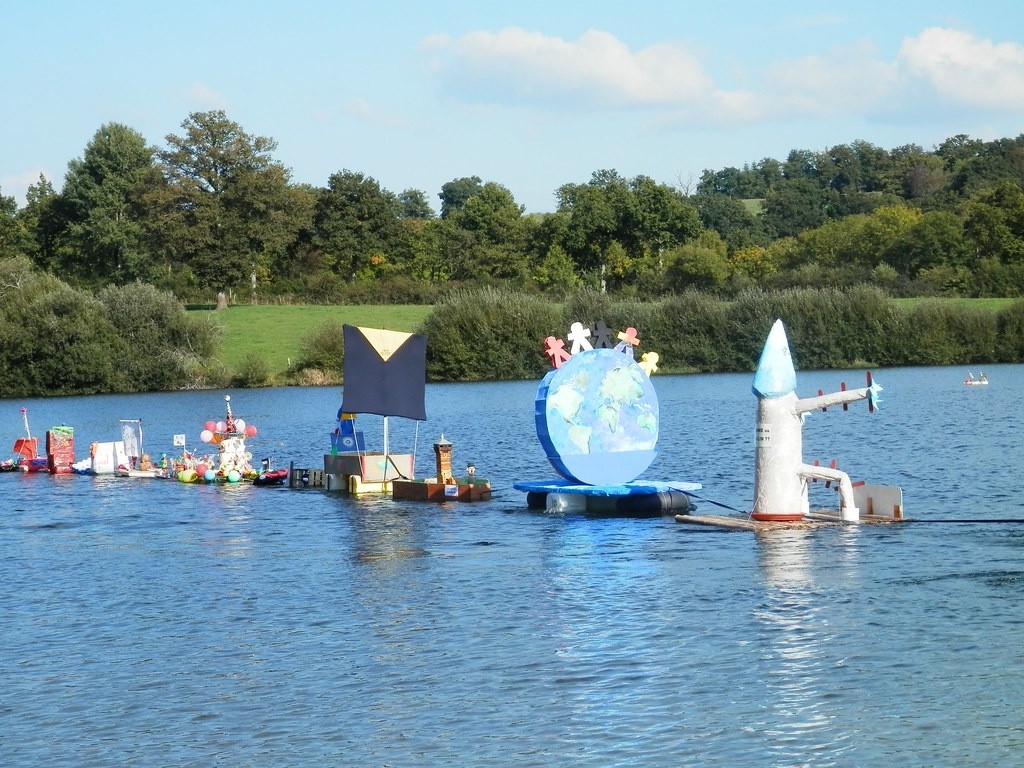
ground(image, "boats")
xmin=963 ymin=369 xmax=989 ymax=385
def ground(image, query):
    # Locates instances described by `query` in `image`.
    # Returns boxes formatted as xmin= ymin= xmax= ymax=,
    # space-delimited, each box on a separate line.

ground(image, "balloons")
xmin=234 ymin=418 xmax=246 ymax=432
xmin=182 ymin=470 xmax=197 ymax=482
xmin=204 ymin=470 xmax=215 ymax=480
xmin=200 ymin=430 xmax=226 ymax=444
xmin=229 ymin=470 xmax=240 ymax=482
xmin=216 ymin=421 xmax=227 ymax=431
xmin=196 ymin=464 xmax=207 ymax=477
xmin=205 ymin=421 xmax=216 ymax=432
xmin=245 ymin=425 xmax=257 ymax=437
xmin=177 ymin=471 xmax=186 ymax=482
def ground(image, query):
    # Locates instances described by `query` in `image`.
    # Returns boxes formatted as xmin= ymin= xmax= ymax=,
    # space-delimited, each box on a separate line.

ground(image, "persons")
xmin=202 ymin=455 xmax=213 ymax=470
